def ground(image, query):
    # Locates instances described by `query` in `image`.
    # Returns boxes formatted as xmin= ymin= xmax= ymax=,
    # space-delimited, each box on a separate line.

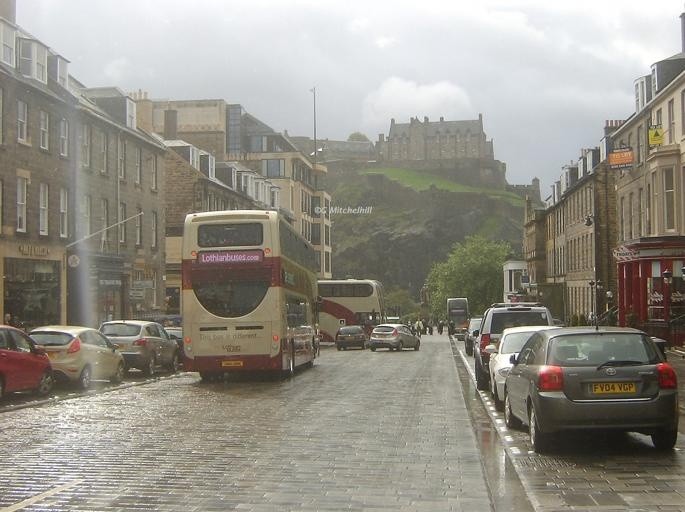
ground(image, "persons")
xmin=415 ymin=317 xmax=455 ymax=336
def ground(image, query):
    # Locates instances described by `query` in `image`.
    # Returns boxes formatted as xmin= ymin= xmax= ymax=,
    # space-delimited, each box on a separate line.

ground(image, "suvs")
xmin=369 ymin=323 xmax=420 ymax=352
xmin=101 ymin=318 xmax=180 ymax=378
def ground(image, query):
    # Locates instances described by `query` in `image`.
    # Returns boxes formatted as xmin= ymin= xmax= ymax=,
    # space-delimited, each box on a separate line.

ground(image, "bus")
xmin=179 ymin=210 xmax=322 ymax=385
xmin=445 ymin=297 xmax=469 ymax=332
xmin=316 ymin=275 xmax=386 ymax=343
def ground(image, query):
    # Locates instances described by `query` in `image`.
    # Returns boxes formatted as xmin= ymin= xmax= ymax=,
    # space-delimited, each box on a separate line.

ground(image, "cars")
xmin=336 ymin=326 xmax=369 ymax=350
xmin=165 ymin=326 xmax=183 ymax=357
xmin=288 ymin=323 xmax=319 ymax=353
xmin=464 ymin=301 xmax=679 ymax=457
xmin=0 ymin=323 xmax=52 ymax=396
xmin=27 ymin=325 xmax=126 ymax=390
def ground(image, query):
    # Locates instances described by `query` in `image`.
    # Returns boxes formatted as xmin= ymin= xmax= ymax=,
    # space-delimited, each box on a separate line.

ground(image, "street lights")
xmin=589 ymin=278 xmax=603 ymax=330
xmin=604 ymin=292 xmax=613 ymax=309
xmin=681 ymin=267 xmax=685 ymax=282
xmin=661 ymin=270 xmax=676 ymax=349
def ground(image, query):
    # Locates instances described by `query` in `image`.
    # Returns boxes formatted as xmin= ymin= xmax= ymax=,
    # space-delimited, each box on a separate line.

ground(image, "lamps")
xmin=584 ymin=214 xmax=594 ymax=227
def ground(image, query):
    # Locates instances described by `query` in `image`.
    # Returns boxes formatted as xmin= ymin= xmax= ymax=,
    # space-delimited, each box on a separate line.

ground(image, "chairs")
xmin=603 ymin=341 xmax=634 ymax=360
xmin=552 ymin=345 xmax=579 ymax=362
